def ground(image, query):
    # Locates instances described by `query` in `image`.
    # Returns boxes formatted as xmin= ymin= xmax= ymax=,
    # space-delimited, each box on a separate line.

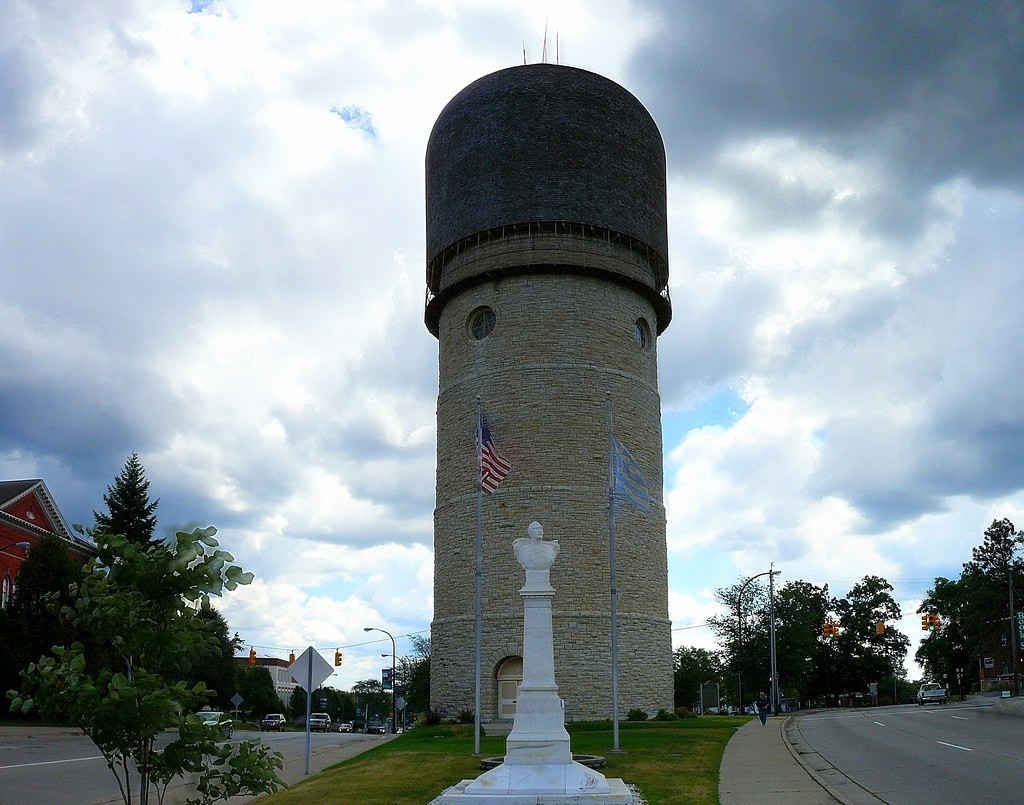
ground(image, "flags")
xmin=613 ymin=435 xmax=650 ymax=514
xmin=476 ymin=414 xmax=512 ymax=496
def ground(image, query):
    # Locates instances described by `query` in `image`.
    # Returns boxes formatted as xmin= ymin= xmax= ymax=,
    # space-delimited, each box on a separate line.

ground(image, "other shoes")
xmin=762 ymin=723 xmax=765 ymax=727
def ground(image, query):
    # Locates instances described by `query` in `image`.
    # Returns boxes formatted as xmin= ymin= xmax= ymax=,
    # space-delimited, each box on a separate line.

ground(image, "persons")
xmin=741 ymin=704 xmax=745 ymax=716
xmin=756 ymin=692 xmax=769 ymax=727
xmin=774 ymin=704 xmax=779 ymax=716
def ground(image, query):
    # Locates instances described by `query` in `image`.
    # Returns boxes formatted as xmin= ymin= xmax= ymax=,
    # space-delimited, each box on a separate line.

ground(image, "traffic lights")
xmin=821 ymin=623 xmax=840 ymax=639
xmin=249 ymin=649 xmax=257 ymax=666
xmin=334 ymin=652 xmax=342 ymax=667
xmin=921 ymin=615 xmax=939 ymax=632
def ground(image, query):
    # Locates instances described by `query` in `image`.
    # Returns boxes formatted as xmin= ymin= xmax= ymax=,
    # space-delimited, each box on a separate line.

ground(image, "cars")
xmin=366 ymin=722 xmax=414 ymax=734
xmin=338 ymin=724 xmax=353 ymax=733
xmin=193 ymin=710 xmax=234 ymax=740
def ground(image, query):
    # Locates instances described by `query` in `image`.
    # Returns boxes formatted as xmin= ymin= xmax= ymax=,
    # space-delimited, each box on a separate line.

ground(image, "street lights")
xmin=381 ymin=653 xmax=406 ymax=734
xmin=363 ymin=627 xmax=396 ymax=734
xmin=738 ymin=570 xmax=783 ymax=716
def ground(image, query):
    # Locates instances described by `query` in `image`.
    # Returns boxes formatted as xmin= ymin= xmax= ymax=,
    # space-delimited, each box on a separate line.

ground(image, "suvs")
xmin=305 ymin=712 xmax=331 ymax=733
xmin=261 ymin=713 xmax=287 ymax=733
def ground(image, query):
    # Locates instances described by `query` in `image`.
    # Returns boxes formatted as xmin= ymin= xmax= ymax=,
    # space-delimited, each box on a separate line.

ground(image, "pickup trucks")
xmin=916 ymin=683 xmax=948 ymax=706
